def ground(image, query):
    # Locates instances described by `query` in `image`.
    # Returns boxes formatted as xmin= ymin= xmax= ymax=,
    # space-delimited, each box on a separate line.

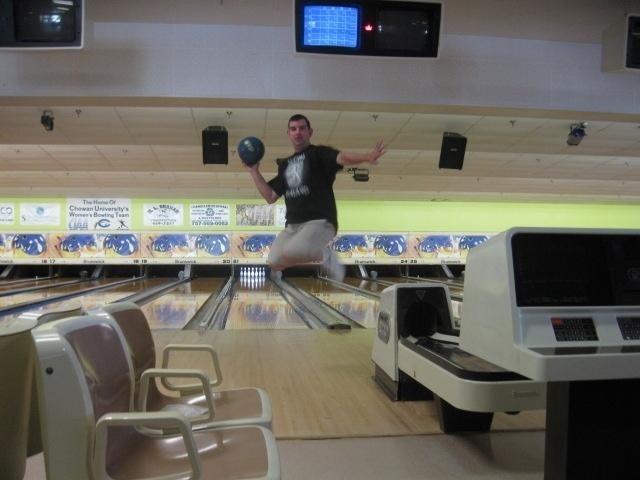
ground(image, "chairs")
xmin=1 ymin=299 xmax=285 ymax=480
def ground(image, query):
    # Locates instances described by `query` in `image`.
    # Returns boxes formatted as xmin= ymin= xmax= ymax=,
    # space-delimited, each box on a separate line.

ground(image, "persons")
xmin=238 ymin=113 xmax=387 ymax=282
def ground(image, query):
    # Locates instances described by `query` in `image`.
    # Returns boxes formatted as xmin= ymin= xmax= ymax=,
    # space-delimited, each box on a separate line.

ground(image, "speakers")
xmin=201 ymin=124 xmax=228 ymax=166
xmin=438 ymin=129 xmax=467 ymax=171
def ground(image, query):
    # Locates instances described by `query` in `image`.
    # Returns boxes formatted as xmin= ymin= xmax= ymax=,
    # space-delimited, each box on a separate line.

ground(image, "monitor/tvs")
xmin=0 ymin=0 xmax=87 ymax=51
xmin=292 ymin=1 xmax=445 ymax=61
